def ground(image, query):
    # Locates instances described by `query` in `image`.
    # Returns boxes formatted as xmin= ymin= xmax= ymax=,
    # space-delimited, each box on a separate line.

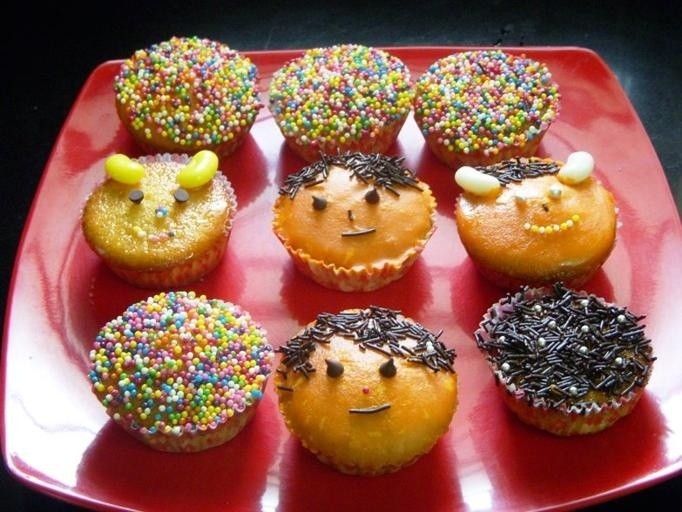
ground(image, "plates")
xmin=4 ymin=44 xmax=680 ymax=511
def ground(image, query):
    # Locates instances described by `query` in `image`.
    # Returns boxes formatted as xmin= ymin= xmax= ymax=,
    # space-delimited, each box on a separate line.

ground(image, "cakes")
xmin=113 ymin=36 xmax=264 ymax=161
xmin=80 ymin=149 xmax=238 ymax=291
xmin=272 ymin=148 xmax=438 ymax=293
xmin=414 ymin=49 xmax=562 ymax=170
xmin=268 ymin=43 xmax=415 ymax=164
xmin=475 ymin=284 xmax=652 ymax=438
xmin=87 ymin=290 xmax=274 ymax=454
xmin=454 ymin=150 xmax=620 ymax=294
xmin=272 ymin=303 xmax=459 ymax=476
